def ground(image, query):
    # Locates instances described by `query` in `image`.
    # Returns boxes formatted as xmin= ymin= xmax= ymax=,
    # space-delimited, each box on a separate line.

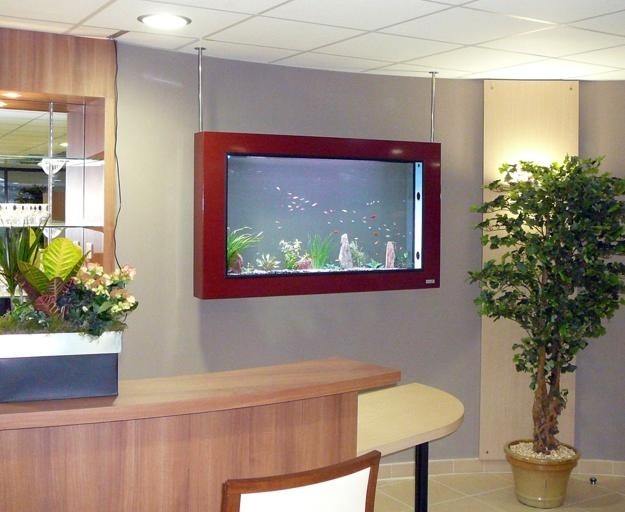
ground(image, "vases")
xmin=0 ymin=332 xmax=122 ymax=401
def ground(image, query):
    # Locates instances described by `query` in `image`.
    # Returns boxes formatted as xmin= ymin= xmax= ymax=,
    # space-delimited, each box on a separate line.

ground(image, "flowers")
xmin=0 ymin=213 xmax=138 ymax=338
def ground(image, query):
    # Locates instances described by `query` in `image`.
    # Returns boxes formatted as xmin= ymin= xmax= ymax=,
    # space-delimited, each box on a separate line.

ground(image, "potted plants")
xmin=462 ymin=154 xmax=624 ymax=509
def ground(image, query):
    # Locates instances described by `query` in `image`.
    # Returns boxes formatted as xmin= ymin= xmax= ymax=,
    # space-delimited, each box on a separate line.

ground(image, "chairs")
xmin=220 ymin=450 xmax=382 ymax=512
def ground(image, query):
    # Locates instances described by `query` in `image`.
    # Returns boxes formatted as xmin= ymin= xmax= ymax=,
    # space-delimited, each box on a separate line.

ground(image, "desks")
xmin=356 ymin=383 xmax=464 ymax=512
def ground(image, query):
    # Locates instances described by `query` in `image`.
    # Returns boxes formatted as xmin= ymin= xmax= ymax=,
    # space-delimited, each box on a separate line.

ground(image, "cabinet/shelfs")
xmin=0 ymin=91 xmax=110 ymax=298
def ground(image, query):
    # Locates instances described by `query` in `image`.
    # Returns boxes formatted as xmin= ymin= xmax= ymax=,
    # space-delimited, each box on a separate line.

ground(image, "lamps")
xmin=137 ymin=12 xmax=192 ymax=33
